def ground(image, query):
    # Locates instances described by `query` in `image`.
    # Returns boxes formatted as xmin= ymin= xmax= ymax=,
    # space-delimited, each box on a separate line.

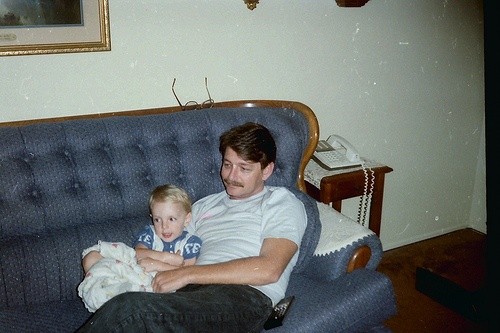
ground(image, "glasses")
xmin=172 ymin=77 xmax=214 ymax=111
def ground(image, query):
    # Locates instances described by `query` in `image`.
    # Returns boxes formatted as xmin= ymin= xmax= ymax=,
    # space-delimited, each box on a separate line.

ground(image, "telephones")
xmin=311 ymin=134 xmax=366 ymax=172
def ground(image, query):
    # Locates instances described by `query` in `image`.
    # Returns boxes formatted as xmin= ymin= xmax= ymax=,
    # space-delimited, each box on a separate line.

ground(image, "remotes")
xmin=263 ymin=295 xmax=295 ymax=330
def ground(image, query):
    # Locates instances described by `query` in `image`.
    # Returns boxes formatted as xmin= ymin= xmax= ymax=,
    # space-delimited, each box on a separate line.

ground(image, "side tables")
xmin=304 ymin=157 xmax=393 ymax=237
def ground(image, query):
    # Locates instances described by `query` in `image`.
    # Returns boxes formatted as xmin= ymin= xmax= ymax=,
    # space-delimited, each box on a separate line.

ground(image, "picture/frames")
xmin=0 ymin=0 xmax=111 ymax=56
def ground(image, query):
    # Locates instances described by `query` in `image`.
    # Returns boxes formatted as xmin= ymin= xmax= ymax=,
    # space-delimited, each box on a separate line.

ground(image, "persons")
xmin=82 ymin=185 xmax=200 ymax=291
xmin=75 ymin=123 xmax=307 ymax=332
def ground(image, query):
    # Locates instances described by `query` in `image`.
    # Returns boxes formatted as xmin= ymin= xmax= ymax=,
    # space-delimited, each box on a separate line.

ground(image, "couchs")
xmin=0 ymin=100 xmax=397 ymax=333
